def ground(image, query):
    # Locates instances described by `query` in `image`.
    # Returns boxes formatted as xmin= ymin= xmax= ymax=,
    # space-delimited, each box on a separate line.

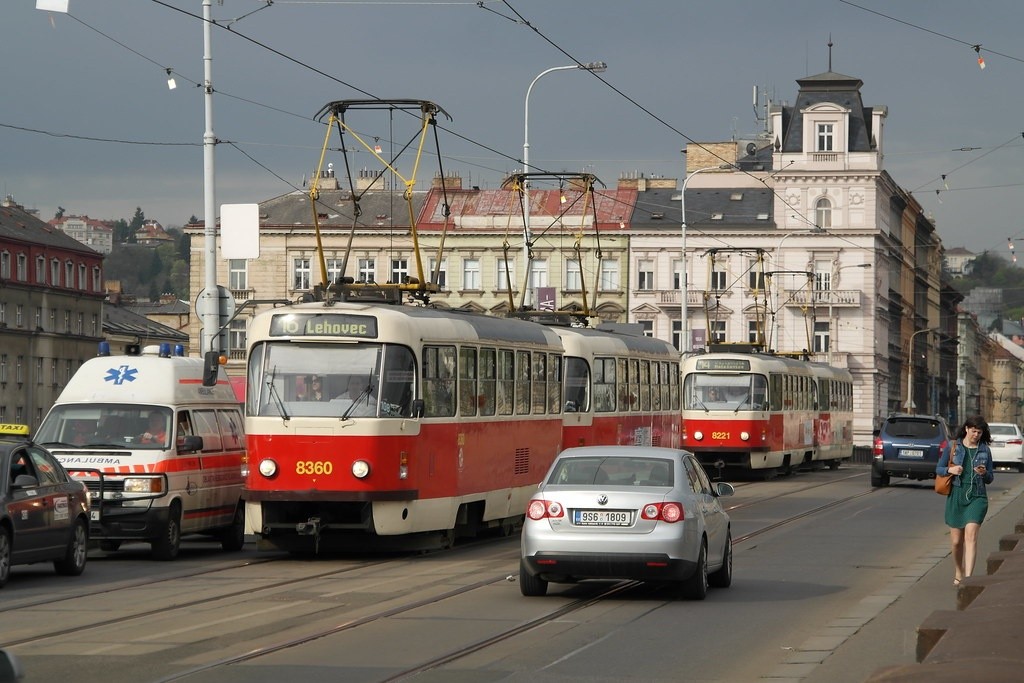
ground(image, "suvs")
xmin=872 ymin=415 xmax=955 ymax=486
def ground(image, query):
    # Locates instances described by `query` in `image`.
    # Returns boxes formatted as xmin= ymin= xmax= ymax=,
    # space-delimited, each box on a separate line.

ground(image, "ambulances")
xmin=18 ymin=341 xmax=247 ymax=559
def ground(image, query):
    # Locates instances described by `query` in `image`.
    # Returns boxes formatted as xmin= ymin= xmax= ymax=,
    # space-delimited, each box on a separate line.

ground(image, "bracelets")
xmin=151 ymin=435 xmax=154 ymax=441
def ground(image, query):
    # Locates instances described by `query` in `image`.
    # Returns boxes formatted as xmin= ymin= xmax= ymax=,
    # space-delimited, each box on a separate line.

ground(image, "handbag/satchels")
xmin=935 ymin=440 xmax=956 ymax=495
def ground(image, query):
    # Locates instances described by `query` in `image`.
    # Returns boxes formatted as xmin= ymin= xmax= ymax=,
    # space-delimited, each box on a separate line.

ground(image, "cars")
xmin=519 ymin=445 xmax=736 ymax=601
xmin=987 ymin=423 xmax=1024 ymax=468
xmin=0 ymin=423 xmax=93 ymax=585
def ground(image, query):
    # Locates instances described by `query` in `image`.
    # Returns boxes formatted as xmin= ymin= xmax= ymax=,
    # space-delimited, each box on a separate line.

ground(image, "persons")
xmin=707 ymin=389 xmax=719 ymax=402
xmin=136 ymin=411 xmax=186 ymax=445
xmin=936 ymin=415 xmax=994 ymax=589
xmin=303 ymin=375 xmax=377 ymax=406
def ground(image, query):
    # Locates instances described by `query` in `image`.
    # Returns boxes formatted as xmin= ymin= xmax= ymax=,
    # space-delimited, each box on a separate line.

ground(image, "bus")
xmin=202 ymin=289 xmax=683 ymax=555
xmin=682 ymin=345 xmax=855 ymax=475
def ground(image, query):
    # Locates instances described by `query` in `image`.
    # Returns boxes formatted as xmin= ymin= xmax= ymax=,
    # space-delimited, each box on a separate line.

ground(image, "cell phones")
xmin=977 ymin=466 xmax=985 ymax=469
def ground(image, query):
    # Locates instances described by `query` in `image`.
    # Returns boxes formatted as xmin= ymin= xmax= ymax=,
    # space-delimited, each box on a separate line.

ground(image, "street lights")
xmin=934 ymin=336 xmax=960 ymax=415
xmin=680 ymin=164 xmax=737 ymax=351
xmin=985 ymin=382 xmax=1011 ymax=422
xmin=827 ymin=264 xmax=873 ymax=365
xmin=908 ymin=326 xmax=941 ymax=415
xmin=524 ymin=60 xmax=607 ymax=307
xmin=774 ymin=229 xmax=825 ymax=351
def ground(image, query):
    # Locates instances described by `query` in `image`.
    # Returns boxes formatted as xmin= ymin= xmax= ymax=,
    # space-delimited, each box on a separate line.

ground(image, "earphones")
xmin=966 ymin=429 xmax=968 ymax=431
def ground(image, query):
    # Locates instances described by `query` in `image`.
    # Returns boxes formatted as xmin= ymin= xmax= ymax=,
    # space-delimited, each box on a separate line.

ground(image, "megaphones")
xmin=125 ymin=345 xmax=140 ymax=356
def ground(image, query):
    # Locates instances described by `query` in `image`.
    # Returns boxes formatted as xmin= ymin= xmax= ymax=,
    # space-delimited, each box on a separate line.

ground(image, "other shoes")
xmin=953 ymin=578 xmax=962 ymax=586
